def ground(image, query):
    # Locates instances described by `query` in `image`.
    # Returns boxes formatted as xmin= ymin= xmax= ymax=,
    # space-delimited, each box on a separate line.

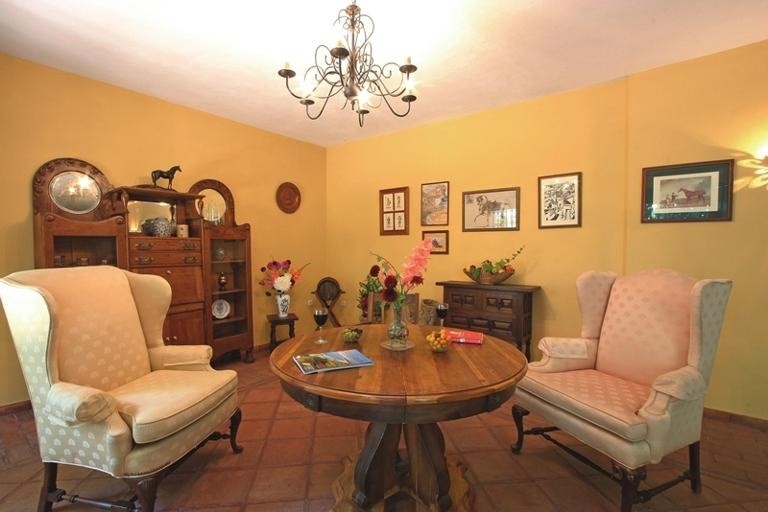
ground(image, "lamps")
xmin=278 ymin=1 xmax=417 ymax=128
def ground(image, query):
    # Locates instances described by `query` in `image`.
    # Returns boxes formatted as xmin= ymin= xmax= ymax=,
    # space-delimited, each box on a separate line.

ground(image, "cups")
xmin=176 ymin=224 xmax=189 ymax=238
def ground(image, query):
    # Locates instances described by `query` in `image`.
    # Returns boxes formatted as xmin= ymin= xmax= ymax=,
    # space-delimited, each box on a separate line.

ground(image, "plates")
xmin=211 ymin=299 xmax=230 ymax=319
xmin=275 ymin=182 xmax=302 ymax=214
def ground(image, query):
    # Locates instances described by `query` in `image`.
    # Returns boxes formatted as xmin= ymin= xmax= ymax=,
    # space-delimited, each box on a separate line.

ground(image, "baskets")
xmin=463 ymin=268 xmax=514 ymax=286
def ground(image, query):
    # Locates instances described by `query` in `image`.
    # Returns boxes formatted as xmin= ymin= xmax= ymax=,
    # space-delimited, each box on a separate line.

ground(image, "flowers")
xmin=258 ymin=258 xmax=311 ymax=296
xmin=369 ymin=236 xmax=432 ymax=304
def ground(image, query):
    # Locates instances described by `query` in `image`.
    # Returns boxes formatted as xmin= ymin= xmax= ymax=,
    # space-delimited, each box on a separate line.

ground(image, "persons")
xmin=664 ymin=192 xmax=678 ymax=208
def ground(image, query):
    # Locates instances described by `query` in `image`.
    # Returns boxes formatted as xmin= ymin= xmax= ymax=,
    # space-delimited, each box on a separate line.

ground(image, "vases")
xmin=386 ymin=295 xmax=408 ymax=348
xmin=275 ymin=293 xmax=290 ymax=318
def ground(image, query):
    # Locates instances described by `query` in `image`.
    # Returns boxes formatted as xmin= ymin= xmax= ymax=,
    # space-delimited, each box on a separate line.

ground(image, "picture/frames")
xmin=461 ymin=186 xmax=519 ymax=232
xmin=640 ymin=158 xmax=735 ymax=224
xmin=421 ymin=229 xmax=449 ymax=255
xmin=379 ymin=186 xmax=409 ymax=235
xmin=420 ymin=181 xmax=449 ymax=226
xmin=538 ymin=171 xmax=582 ymax=229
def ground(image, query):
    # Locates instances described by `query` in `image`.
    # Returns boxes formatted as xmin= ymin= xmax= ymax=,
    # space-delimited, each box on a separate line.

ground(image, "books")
xmin=449 ymin=330 xmax=483 ymax=345
xmin=292 ymin=349 xmax=374 ymax=375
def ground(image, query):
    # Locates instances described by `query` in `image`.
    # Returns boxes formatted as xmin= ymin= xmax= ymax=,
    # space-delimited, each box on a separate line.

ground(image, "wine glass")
xmin=312 ymin=307 xmax=328 ymax=345
xmin=436 ymin=302 xmax=449 ymax=328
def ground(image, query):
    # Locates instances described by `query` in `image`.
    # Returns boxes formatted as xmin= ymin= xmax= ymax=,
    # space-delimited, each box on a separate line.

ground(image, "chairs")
xmin=510 ymin=265 xmax=733 ymax=510
xmin=0 ymin=265 xmax=241 ymax=511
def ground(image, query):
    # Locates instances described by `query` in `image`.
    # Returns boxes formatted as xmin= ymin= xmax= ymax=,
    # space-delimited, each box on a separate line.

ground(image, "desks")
xmin=435 ymin=279 xmax=541 ymax=363
xmin=269 ymin=323 xmax=528 ymax=511
xmin=267 ymin=313 xmax=299 ymax=354
